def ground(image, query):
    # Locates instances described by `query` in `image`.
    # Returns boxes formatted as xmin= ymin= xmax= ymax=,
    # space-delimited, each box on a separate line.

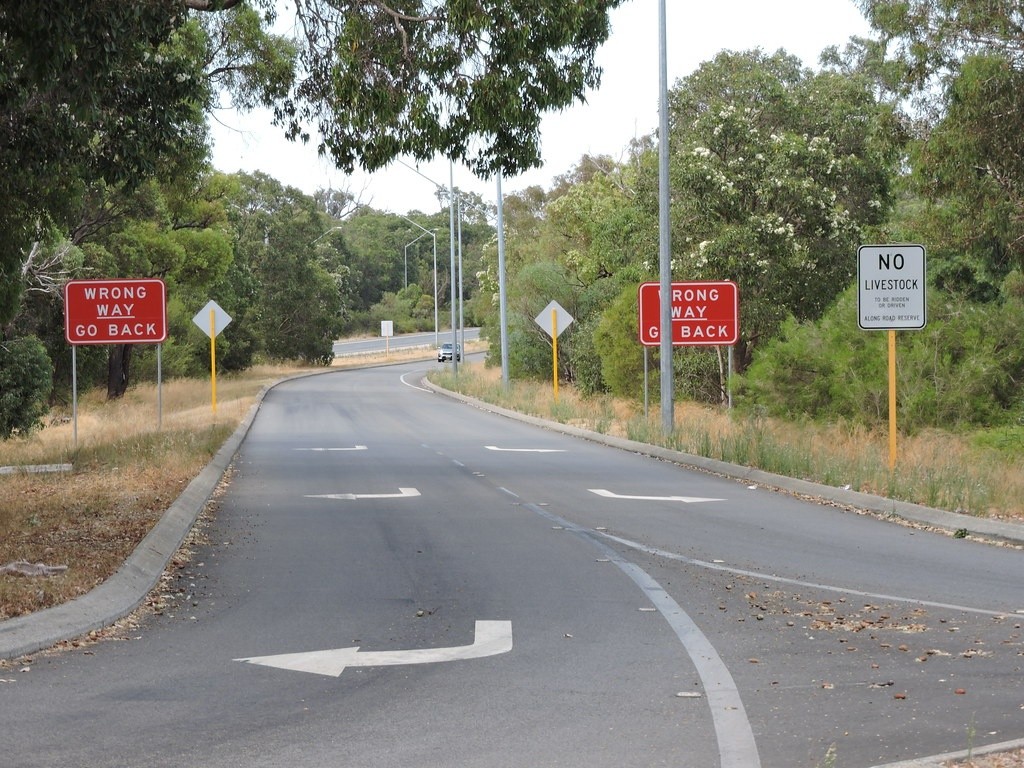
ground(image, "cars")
xmin=437 ymin=343 xmax=460 ymax=363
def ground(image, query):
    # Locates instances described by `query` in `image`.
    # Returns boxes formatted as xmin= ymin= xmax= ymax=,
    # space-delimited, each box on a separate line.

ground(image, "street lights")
xmin=403 ymin=227 xmax=439 ymax=291
xmin=383 ymin=211 xmax=438 ymax=350
